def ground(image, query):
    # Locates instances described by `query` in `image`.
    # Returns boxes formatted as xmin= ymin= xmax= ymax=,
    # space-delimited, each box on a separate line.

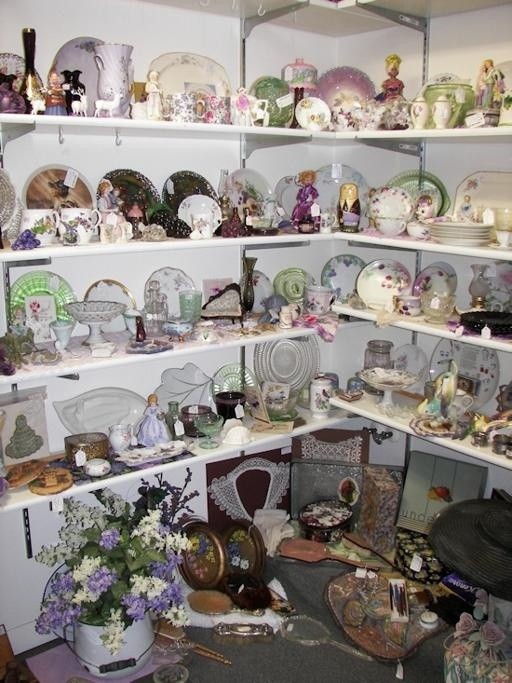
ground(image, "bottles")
xmin=240 ymin=256 xmax=258 ymax=310
xmin=142 ymin=280 xmax=169 ymax=336
xmin=218 ymin=169 xmax=229 ymax=197
xmin=278 ymin=304 xmax=293 ymax=328
xmin=411 ymin=94 xmax=428 ymax=130
xmin=164 ymin=399 xmax=186 ymax=444
xmin=232 ymin=206 xmax=253 ymax=236
xmin=431 ymin=94 xmax=451 ymax=131
xmin=127 ymin=203 xmax=145 ymax=241
xmin=363 ymin=340 xmax=394 ymax=396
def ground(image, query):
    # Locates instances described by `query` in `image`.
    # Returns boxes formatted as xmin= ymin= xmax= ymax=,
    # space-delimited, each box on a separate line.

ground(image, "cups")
xmin=301 ymin=285 xmax=336 ymax=319
xmin=19 ymin=204 xmax=104 ymax=250
xmin=160 ymin=93 xmax=231 ymax=124
xmin=175 ymin=287 xmax=208 ymax=324
xmin=472 ymin=431 xmax=487 ymax=446
xmin=318 ymin=212 xmax=337 ymax=234
xmin=375 ymin=217 xmax=407 ymax=237
xmin=108 ymin=424 xmax=133 ymax=454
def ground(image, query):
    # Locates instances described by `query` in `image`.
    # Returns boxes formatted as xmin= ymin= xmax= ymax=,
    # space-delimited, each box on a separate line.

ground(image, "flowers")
xmin=24 ymin=467 xmax=203 ymax=637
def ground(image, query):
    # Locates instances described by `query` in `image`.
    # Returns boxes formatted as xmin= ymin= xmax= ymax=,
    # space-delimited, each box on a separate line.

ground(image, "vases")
xmin=68 ymin=615 xmax=155 ymax=677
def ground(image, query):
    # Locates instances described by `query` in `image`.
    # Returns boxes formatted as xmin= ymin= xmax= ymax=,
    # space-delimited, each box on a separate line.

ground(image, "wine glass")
xmin=62 ymin=294 xmax=132 ymax=350
xmin=419 ymin=286 xmax=462 ymax=327
xmin=357 ymin=364 xmax=420 ymax=415
xmin=191 ymin=411 xmax=226 ymax=450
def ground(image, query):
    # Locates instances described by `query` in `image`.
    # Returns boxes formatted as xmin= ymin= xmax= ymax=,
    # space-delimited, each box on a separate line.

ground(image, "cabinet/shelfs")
xmin=1 ymin=107 xmax=512 ymax=514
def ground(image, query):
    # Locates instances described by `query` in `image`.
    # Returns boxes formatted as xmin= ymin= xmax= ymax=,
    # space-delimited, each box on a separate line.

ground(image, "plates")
xmin=3 ymin=330 xmax=512 ymax=487
xmin=3 ymin=151 xmax=512 ymax=256
xmin=219 ymin=518 xmax=273 ymax=591
xmin=188 ymin=589 xmax=232 ymax=616
xmin=6 ymin=259 xmax=512 ymax=368
xmin=4 ymin=43 xmax=511 ymax=135
xmin=177 ymin=520 xmax=227 ymax=589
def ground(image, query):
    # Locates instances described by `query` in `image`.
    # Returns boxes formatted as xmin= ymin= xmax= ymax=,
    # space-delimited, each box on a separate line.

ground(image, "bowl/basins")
xmin=393 ymin=295 xmax=420 ymax=316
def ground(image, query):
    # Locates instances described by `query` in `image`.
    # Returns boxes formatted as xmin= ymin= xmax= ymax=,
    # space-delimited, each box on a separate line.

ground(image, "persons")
xmin=291 ymin=170 xmax=320 ymax=231
xmin=97 ymin=182 xmax=132 ymax=242
xmin=380 ymin=53 xmax=408 ymax=130
xmin=45 ymin=73 xmax=68 ymax=116
xmin=144 ymin=70 xmax=162 ymax=120
xmin=337 ymin=184 xmax=360 ymax=232
xmin=478 ymin=60 xmax=503 ymax=105
xmin=135 ymin=395 xmax=172 ymax=447
